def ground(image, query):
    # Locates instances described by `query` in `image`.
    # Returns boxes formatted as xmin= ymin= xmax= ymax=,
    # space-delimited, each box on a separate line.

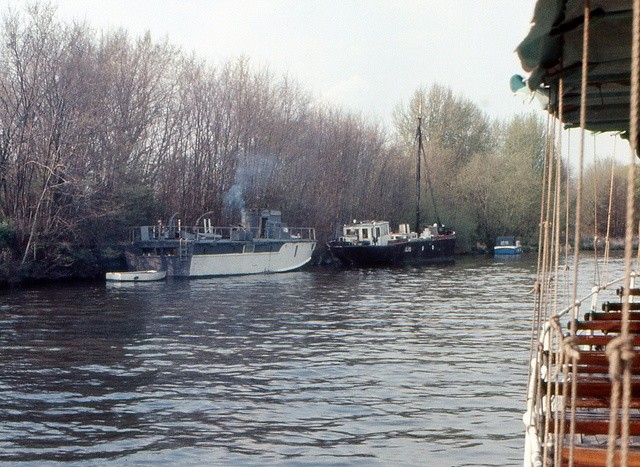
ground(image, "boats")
xmin=124 ymin=209 xmax=317 ymax=279
xmin=494 ymin=237 xmax=524 ymax=255
xmin=106 ymin=270 xmax=168 ymax=282
xmin=327 ymin=220 xmax=457 ymax=270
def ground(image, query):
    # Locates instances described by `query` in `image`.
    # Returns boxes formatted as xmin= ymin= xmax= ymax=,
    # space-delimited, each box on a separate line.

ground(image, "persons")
xmin=475 ymin=239 xmax=489 ymax=254
